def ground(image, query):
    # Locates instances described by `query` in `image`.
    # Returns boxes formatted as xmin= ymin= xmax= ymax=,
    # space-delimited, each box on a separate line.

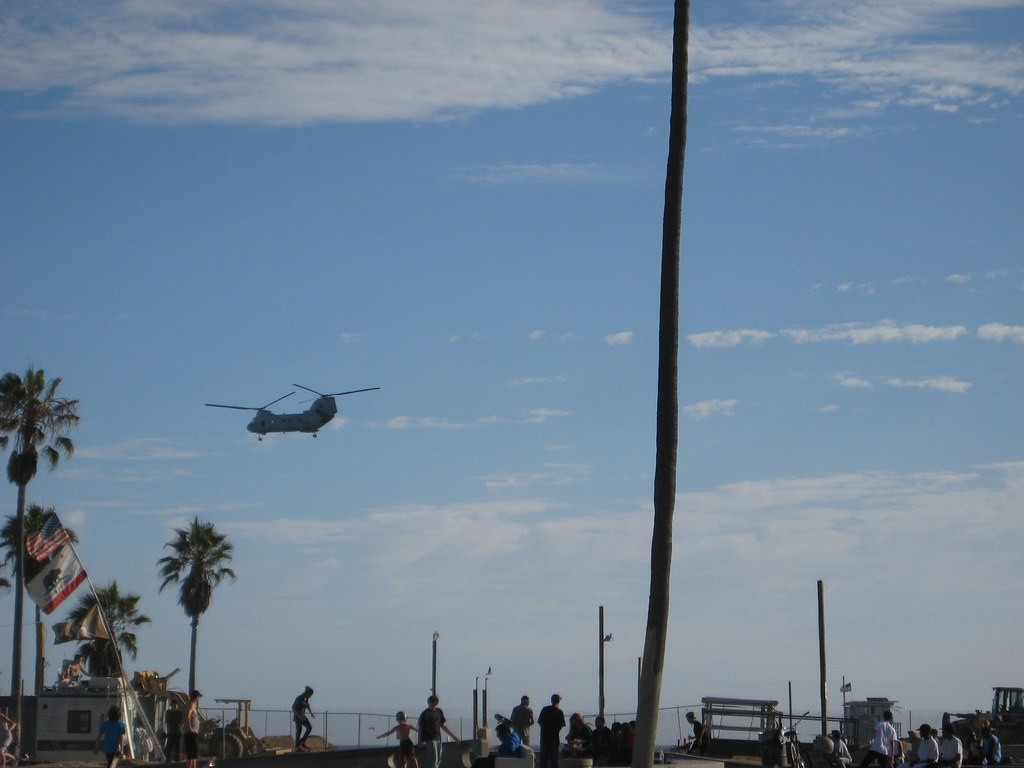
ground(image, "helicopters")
xmin=204 ymin=382 xmax=380 ymax=441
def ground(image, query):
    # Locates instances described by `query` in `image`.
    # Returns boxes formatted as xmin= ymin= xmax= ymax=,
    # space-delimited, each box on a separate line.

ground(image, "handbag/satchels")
xmin=982 ymin=757 xmax=988 ymax=768
xmin=569 ymin=741 xmax=582 ymax=750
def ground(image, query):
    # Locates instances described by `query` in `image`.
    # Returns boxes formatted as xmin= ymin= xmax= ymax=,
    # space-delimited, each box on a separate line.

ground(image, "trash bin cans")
xmin=758 ymin=731 xmax=787 ymax=766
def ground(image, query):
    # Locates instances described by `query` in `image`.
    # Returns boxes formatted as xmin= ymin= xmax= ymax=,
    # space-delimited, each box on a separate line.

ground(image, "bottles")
xmin=983 ymin=758 xmax=988 ymax=768
xmin=659 ymin=750 xmax=664 ymax=764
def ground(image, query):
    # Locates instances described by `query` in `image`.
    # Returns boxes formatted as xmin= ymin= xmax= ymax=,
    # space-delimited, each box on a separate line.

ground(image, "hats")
xmin=940 ymin=724 xmax=954 ymax=730
xmin=829 ymin=730 xmax=840 ymax=734
xmin=686 ymin=712 xmax=696 ymax=718
xmin=916 ymin=724 xmax=932 ymax=731
xmin=552 ymin=694 xmax=561 ymax=700
xmin=190 ymin=690 xmax=202 ymax=696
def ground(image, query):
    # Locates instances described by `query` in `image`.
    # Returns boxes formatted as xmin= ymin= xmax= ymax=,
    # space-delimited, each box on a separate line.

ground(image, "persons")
xmin=470 ymin=724 xmax=521 ymax=768
xmin=925 ymin=724 xmax=963 ymax=768
xmin=94 ymin=707 xmax=125 ymax=768
xmin=418 ymin=696 xmax=461 ymax=768
xmin=685 ymin=712 xmax=710 ymax=756
xmin=165 ymin=700 xmax=184 ymax=762
xmin=510 ymin=696 xmax=534 ymax=746
xmin=967 ymin=729 xmax=983 ymax=765
xmin=981 ymin=726 xmax=1002 ymax=765
xmin=0 ymin=713 xmax=16 ymax=768
xmin=182 ymin=690 xmax=203 ymax=768
xmin=860 ymin=711 xmax=897 ymax=768
xmin=66 ymin=654 xmax=93 ymax=682
xmin=537 ymin=694 xmax=566 ymax=768
xmin=292 ymin=686 xmax=315 ymax=749
xmin=376 ymin=711 xmax=420 ymax=768
xmin=565 ymin=713 xmax=636 ymax=768
xmin=824 ymin=730 xmax=852 ymax=768
xmin=897 ymin=724 xmax=939 ymax=768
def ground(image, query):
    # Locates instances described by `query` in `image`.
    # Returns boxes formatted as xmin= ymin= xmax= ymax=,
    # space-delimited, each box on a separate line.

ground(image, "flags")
xmin=24 ymin=511 xmax=87 ymax=614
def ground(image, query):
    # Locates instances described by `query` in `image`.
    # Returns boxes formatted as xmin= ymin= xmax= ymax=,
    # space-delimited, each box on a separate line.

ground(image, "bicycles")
xmin=784 ymin=711 xmax=812 ymax=768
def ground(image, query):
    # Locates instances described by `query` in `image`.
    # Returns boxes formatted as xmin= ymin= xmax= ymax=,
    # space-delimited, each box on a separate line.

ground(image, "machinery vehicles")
xmin=941 ymin=686 xmax=1024 ymax=745
xmin=135 ymin=667 xmax=291 ymax=760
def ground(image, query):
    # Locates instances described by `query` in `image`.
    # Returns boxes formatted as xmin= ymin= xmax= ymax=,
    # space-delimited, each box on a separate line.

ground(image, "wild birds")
xmin=433 ymin=630 xmax=439 ymax=640
xmin=486 ymin=666 xmax=494 ymax=675
